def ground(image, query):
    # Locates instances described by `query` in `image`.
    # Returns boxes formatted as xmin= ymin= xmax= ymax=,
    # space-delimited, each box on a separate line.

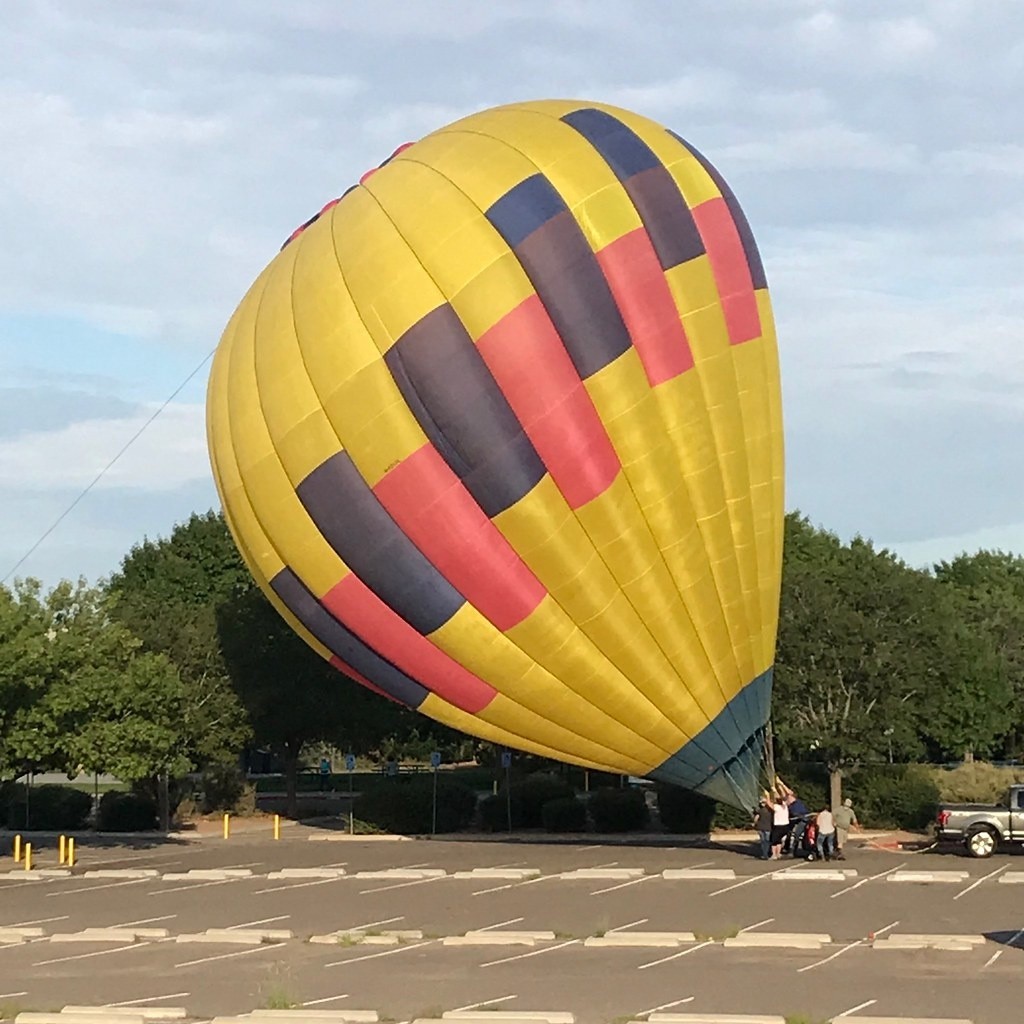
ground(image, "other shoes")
xmin=768 ymin=854 xmax=782 ymax=860
xmin=317 ymin=791 xmax=324 ymax=796
xmin=331 ymin=788 xmax=336 ymax=794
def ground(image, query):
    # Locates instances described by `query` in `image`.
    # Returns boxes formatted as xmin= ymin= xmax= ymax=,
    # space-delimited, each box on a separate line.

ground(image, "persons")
xmin=320 ymin=758 xmax=332 ymax=784
xmin=385 ymin=755 xmax=399 ymax=776
xmin=753 ymin=776 xmax=857 ymax=860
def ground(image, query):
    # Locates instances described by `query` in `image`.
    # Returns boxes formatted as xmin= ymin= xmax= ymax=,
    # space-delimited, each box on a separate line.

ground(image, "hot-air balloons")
xmin=204 ymin=100 xmax=841 ymax=861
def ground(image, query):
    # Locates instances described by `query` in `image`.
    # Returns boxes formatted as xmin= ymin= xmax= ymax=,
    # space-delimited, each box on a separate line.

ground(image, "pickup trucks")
xmin=934 ymin=784 xmax=1024 ymax=858
